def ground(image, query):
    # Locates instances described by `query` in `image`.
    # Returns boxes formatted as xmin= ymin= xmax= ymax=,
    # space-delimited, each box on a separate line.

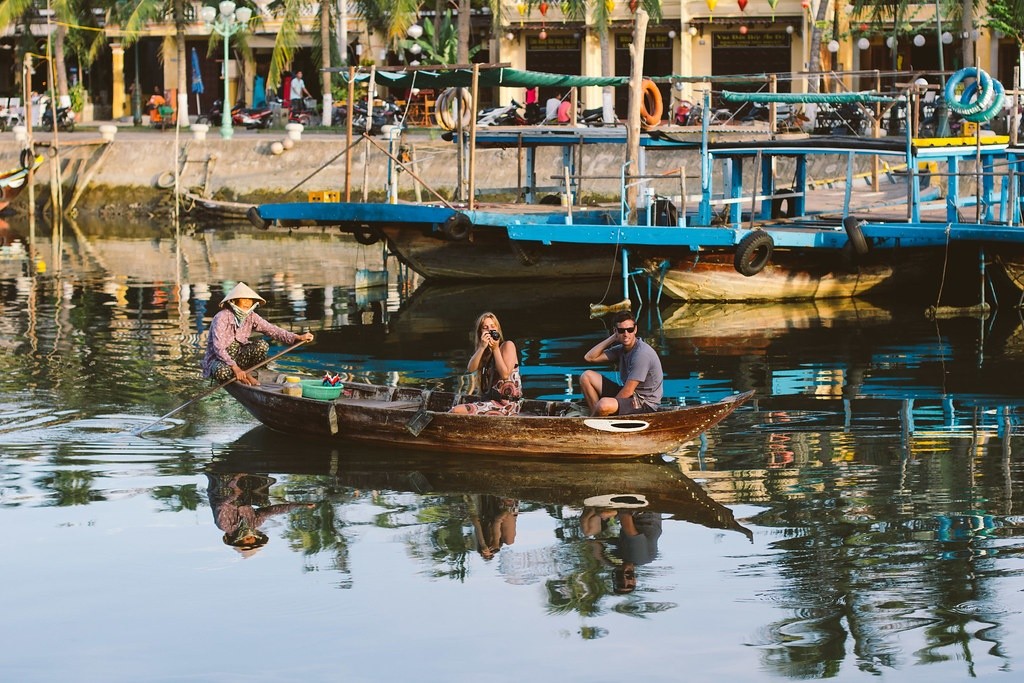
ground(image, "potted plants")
xmin=67 ymin=84 xmax=83 ymax=123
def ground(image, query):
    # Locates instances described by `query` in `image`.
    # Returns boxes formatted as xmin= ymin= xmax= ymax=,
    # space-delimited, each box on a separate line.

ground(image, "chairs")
xmin=0 ymin=98 xmax=23 ymax=126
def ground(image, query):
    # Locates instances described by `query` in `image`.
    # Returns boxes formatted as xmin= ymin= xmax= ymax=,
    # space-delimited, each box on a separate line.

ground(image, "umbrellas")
xmin=191 ymin=48 xmax=204 ymax=116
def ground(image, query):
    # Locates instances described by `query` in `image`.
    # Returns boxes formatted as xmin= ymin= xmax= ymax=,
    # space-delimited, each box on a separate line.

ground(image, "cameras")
xmin=485 ymin=329 xmax=500 ymax=341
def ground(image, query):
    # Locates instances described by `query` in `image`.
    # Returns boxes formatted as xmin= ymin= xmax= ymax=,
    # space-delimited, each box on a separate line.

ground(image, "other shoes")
xmin=294 ymin=114 xmax=299 ymax=117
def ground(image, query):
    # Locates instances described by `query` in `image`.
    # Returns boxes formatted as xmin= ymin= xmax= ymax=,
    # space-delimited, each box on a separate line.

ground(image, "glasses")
xmin=617 ymin=325 xmax=634 ymax=334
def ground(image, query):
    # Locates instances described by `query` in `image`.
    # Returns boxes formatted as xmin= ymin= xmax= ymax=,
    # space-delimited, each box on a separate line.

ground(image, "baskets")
xmin=302 ymin=379 xmax=343 ymax=399
xmin=304 ymin=99 xmax=317 ymax=109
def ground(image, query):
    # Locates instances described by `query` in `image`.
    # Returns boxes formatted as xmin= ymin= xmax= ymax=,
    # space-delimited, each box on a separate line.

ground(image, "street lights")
xmin=204 ymin=1 xmax=252 ymax=138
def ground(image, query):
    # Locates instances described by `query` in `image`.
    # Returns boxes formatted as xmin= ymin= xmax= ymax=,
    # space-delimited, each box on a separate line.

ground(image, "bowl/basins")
xmin=302 ymin=379 xmax=345 ymax=400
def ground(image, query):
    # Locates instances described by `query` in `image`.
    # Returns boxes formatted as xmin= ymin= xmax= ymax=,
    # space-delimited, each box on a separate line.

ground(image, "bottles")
xmin=282 ymin=376 xmax=303 ymax=397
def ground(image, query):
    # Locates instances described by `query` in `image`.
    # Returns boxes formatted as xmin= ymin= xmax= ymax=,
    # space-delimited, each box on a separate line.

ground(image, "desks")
xmin=401 ymin=104 xmax=432 ymax=125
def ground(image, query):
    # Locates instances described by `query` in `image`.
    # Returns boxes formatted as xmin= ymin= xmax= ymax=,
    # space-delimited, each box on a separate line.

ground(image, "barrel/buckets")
xmin=560 ymin=194 xmax=574 ymax=208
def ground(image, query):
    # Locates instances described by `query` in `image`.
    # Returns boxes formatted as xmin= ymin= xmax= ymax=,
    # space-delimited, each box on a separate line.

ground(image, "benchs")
xmin=373 ymin=101 xmax=436 ymax=127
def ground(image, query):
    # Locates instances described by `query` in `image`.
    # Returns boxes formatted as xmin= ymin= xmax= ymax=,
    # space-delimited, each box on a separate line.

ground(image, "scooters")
xmin=146 ymin=96 xmax=410 ymax=135
xmin=41 ymin=96 xmax=76 ymax=133
xmin=671 ymin=92 xmax=963 ymax=137
xmin=477 ymin=95 xmax=611 ymax=129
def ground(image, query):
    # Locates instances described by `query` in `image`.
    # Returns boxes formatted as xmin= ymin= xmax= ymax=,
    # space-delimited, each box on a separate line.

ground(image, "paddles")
xmin=136 ymin=339 xmax=310 ymax=434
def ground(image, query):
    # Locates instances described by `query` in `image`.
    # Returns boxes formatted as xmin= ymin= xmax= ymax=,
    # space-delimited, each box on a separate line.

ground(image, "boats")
xmin=644 ymin=300 xmax=894 ymax=338
xmin=211 ymin=361 xmax=756 ymax=461
xmin=249 ymin=62 xmax=1024 ymax=280
xmin=203 ymin=425 xmax=756 ymax=543
xmin=626 ymin=249 xmax=896 ymax=302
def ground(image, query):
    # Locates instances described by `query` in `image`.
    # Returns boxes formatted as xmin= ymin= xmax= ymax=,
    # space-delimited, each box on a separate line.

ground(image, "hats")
xmin=219 ymin=282 xmax=266 ymax=308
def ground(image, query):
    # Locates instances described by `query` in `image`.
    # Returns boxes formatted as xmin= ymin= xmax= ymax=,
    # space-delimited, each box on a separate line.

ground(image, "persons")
xmin=546 ymin=92 xmax=562 ymax=122
xmin=581 ymin=508 xmax=661 ymax=594
xmin=291 ymin=70 xmax=312 ymax=118
xmin=523 ymin=86 xmax=539 ymax=124
xmin=465 ymin=495 xmax=518 ymax=560
xmin=205 ymin=282 xmax=315 ymax=386
xmin=557 ymin=95 xmax=571 ymax=123
xmin=209 ymin=475 xmax=315 ymax=559
xmin=450 ymin=312 xmax=523 ymax=414
xmin=579 ymin=311 xmax=663 ymax=415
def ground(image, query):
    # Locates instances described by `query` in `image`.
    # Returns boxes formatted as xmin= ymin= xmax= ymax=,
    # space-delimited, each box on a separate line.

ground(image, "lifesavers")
xmin=247 ymin=206 xmax=272 ymax=230
xmin=354 ymin=224 xmax=379 ymax=244
xmin=156 ymin=171 xmax=179 ymax=188
xmin=733 ymin=230 xmax=775 ymax=277
xmin=844 ymin=215 xmax=869 ymax=255
xmin=651 ymin=199 xmax=680 ymax=226
xmin=640 ymin=79 xmax=664 ymax=131
xmin=434 ymin=86 xmax=473 ymax=132
xmin=443 ymin=213 xmax=472 ymax=241
xmin=944 ymin=66 xmax=1006 ymax=123
xmin=508 ymin=240 xmax=545 ymax=266
xmin=20 ymin=149 xmax=34 ymax=170
xmin=772 ymin=188 xmax=795 ymax=218
xmin=539 ymin=195 xmax=561 ymax=205
